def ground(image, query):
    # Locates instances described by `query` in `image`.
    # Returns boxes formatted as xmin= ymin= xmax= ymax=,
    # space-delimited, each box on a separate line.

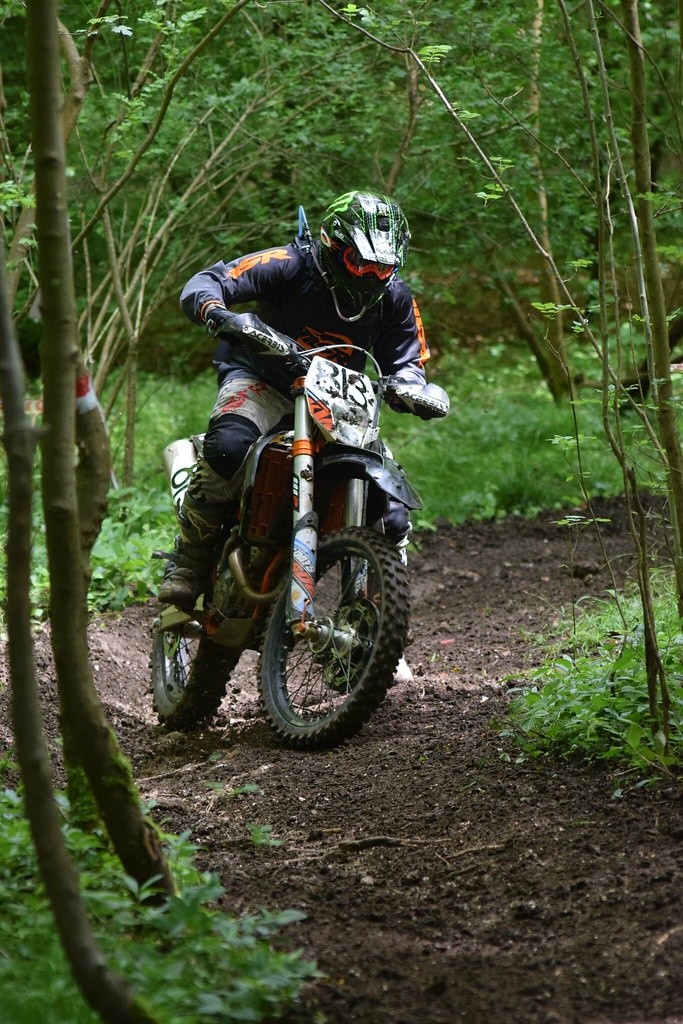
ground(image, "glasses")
xmin=344 ymin=246 xmax=400 ymax=281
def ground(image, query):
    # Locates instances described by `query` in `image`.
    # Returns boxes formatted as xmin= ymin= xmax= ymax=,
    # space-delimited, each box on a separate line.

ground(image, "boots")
xmin=152 ymin=456 xmax=244 ymax=607
xmin=380 ymin=536 xmax=416 ymax=685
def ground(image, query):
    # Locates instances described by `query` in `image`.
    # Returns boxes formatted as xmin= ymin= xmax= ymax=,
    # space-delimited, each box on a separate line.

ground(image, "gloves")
xmin=207 ymin=308 xmax=247 ymax=347
xmin=386 ymin=373 xmax=425 ymax=413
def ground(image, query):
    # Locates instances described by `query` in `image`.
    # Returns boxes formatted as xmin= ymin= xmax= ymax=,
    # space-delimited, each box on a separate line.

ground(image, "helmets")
xmin=320 ymin=190 xmax=411 ymax=312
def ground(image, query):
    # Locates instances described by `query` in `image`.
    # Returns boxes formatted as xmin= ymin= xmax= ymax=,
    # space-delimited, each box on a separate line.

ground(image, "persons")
xmin=158 ymin=191 xmax=447 ymax=680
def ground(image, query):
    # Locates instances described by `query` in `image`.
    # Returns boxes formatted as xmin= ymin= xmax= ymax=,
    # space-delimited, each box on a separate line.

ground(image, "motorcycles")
xmin=145 ymin=312 xmax=449 ymax=752
